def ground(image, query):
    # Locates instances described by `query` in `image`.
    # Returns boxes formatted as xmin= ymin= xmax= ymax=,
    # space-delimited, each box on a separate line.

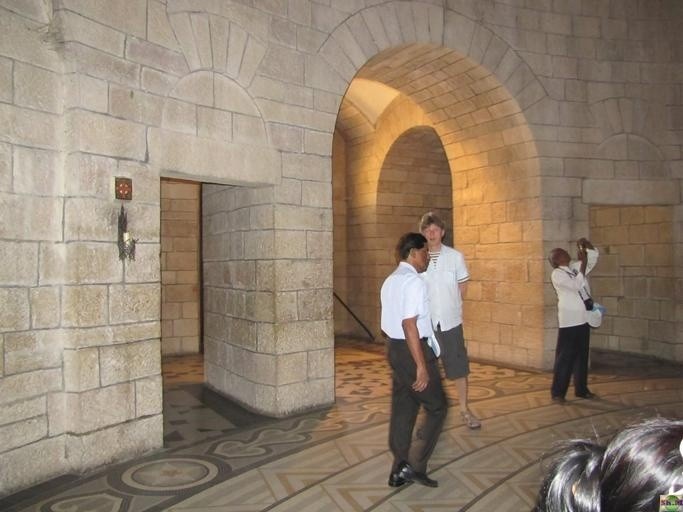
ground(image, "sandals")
xmin=459 ymin=408 xmax=484 ymax=430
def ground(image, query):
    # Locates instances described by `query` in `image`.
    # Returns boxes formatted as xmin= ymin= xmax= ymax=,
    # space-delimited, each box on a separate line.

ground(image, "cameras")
xmin=578 ymin=243 xmax=584 ymax=251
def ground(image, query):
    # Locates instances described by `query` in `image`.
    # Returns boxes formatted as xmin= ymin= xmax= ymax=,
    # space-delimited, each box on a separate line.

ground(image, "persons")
xmin=547 ymin=237 xmax=600 ymax=404
xmin=378 ymin=232 xmax=449 ymax=490
xmin=521 ymin=416 xmax=683 ymax=511
xmin=417 ymin=210 xmax=482 ymax=430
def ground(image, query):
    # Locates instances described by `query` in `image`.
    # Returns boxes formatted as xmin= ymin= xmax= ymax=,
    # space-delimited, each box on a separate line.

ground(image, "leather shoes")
xmin=388 ymin=470 xmax=408 ymax=487
xmin=575 ymin=390 xmax=600 ymax=401
xmin=550 ymin=394 xmax=571 ymax=406
xmin=395 ymin=460 xmax=437 ymax=488
